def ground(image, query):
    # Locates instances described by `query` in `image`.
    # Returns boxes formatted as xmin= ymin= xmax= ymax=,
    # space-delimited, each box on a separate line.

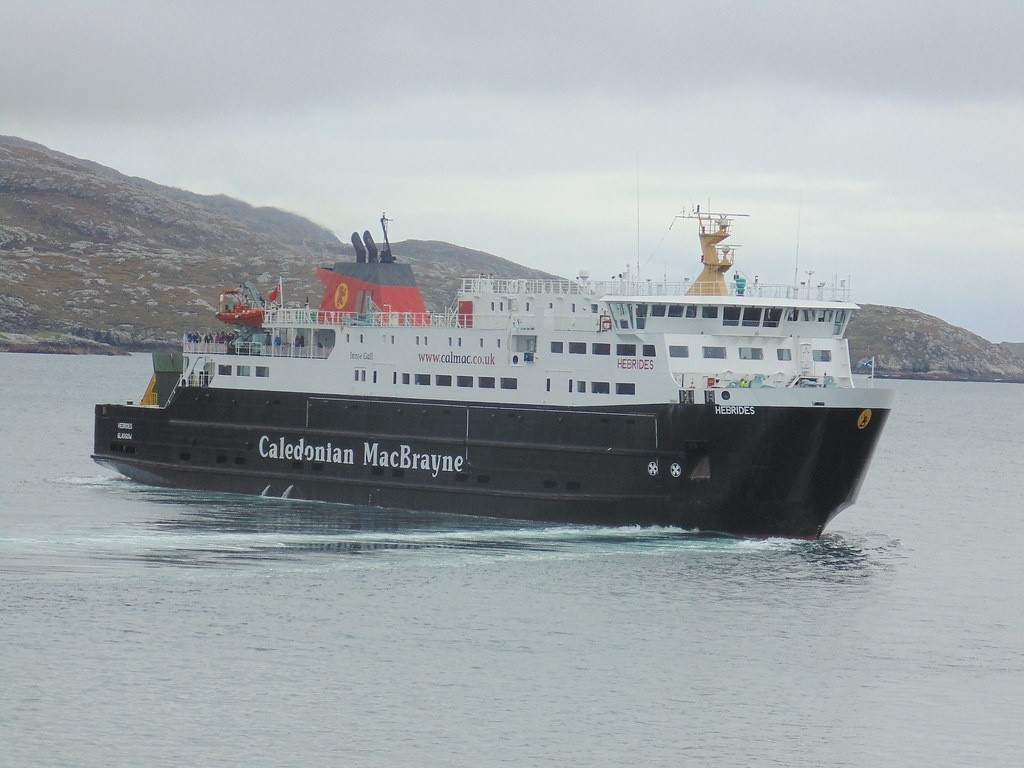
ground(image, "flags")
xmin=270 ymin=284 xmax=280 ymax=302
xmin=862 ymin=360 xmax=872 ymax=368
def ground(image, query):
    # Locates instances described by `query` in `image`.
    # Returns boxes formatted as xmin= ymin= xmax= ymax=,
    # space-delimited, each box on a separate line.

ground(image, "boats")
xmin=214 ymin=308 xmax=268 ymax=328
xmin=90 ymin=194 xmax=900 ymax=545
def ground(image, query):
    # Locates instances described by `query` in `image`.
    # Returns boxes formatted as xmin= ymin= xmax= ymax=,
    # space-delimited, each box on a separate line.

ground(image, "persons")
xmin=274 ymin=335 xmax=281 ymax=355
xmin=187 ymin=331 xmax=240 ymax=355
xmin=294 ymin=335 xmax=304 ymax=358
